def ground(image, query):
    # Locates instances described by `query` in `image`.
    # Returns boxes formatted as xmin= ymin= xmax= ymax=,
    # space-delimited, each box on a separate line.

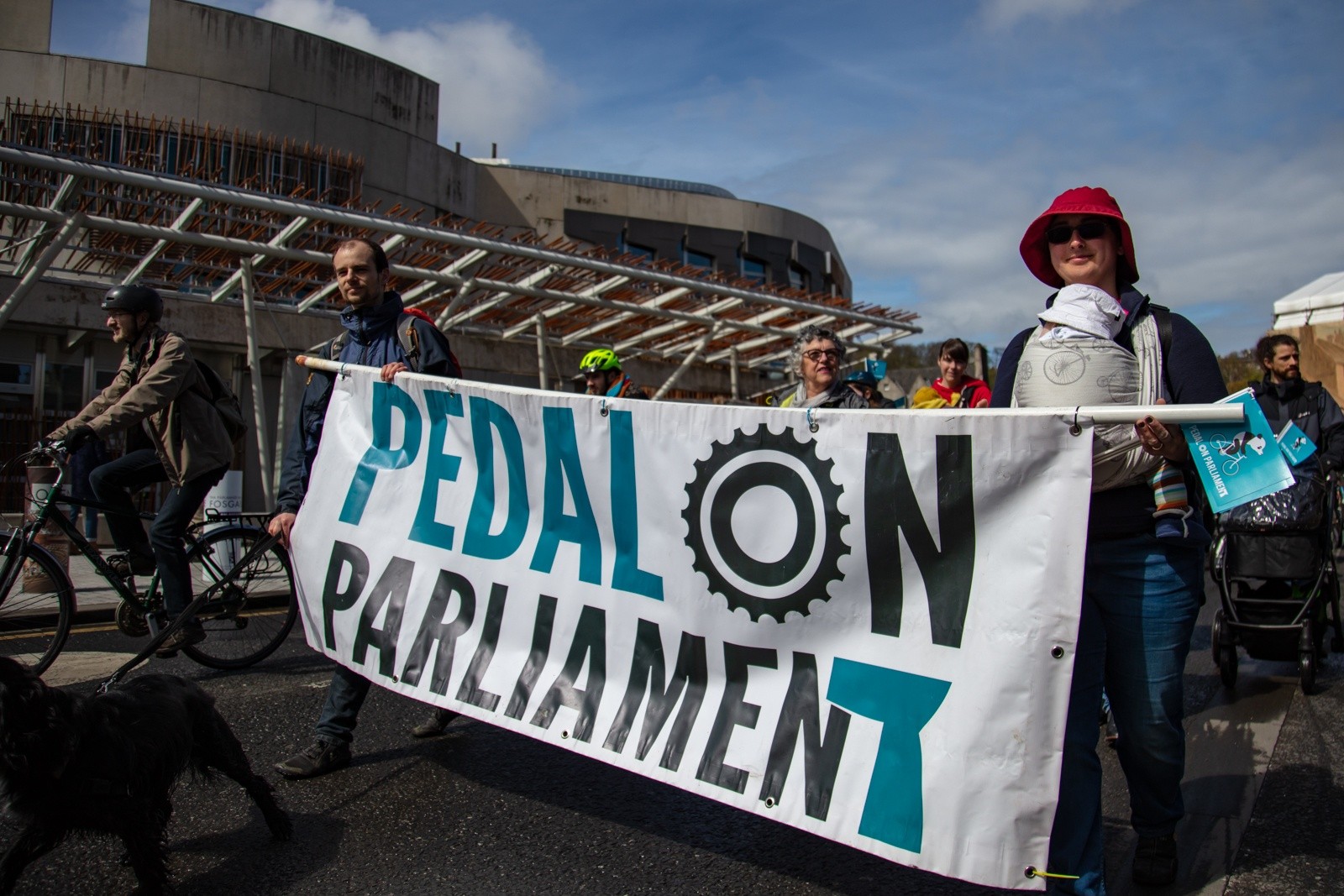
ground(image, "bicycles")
xmin=0 ymin=436 xmax=299 ymax=678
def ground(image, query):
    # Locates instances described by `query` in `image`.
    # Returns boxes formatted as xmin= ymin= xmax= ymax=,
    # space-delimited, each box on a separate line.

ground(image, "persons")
xmin=67 ymin=438 xmax=108 ymax=555
xmin=1254 ymin=334 xmax=1344 ymax=473
xmin=774 ymin=324 xmax=869 ymax=409
xmin=842 ymin=370 xmax=895 ymax=409
xmin=268 ymin=238 xmax=461 ymax=780
xmin=580 ymin=348 xmax=649 ymax=400
xmin=990 ymin=186 xmax=1229 ymax=896
xmin=931 ymin=338 xmax=991 ymax=408
xmin=33 ymin=284 xmax=235 ymax=653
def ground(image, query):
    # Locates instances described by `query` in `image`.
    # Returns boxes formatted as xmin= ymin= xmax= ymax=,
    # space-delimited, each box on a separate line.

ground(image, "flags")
xmin=1179 ymin=393 xmax=1296 ymax=514
xmin=1278 ymin=422 xmax=1317 ymax=466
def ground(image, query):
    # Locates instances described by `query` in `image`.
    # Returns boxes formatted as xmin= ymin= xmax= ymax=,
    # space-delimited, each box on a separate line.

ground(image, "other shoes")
xmin=156 ymin=618 xmax=206 ymax=653
xmin=95 ymin=553 xmax=156 ymax=576
xmin=1131 ymin=836 xmax=1178 ymax=888
xmin=1151 ymin=506 xmax=1212 ymax=550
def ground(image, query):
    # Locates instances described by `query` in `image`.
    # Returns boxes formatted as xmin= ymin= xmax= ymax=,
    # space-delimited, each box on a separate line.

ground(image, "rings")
xmin=1152 ymin=443 xmax=1163 ymax=450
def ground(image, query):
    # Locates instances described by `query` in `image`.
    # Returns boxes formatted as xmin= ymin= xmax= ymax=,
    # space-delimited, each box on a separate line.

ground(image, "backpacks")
xmin=331 ymin=308 xmax=463 ymax=380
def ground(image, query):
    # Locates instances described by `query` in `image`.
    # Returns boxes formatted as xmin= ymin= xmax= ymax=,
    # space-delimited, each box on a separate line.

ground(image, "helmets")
xmin=579 ymin=348 xmax=623 ymax=376
xmin=101 ymin=285 xmax=164 ymax=322
xmin=843 ymin=370 xmax=877 ymax=389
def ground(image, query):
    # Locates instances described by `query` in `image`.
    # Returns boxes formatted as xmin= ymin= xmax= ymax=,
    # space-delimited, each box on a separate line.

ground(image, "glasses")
xmin=801 ymin=349 xmax=839 ymax=361
xmin=1043 ymin=221 xmax=1108 ymax=245
xmin=106 ymin=312 xmax=131 ymax=318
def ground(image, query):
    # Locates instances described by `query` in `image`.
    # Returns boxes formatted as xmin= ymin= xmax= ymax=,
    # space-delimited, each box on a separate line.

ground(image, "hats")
xmin=1020 ymin=186 xmax=1140 ymax=289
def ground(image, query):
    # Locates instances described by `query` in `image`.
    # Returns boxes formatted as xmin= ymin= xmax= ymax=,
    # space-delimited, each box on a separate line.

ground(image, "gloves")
xmin=32 ymin=437 xmax=57 ymax=447
xmin=65 ymin=424 xmax=96 ymax=455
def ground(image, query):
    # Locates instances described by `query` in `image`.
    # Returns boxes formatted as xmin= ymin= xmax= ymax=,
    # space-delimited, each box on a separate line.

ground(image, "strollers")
xmin=1211 ymin=461 xmax=1344 ymax=696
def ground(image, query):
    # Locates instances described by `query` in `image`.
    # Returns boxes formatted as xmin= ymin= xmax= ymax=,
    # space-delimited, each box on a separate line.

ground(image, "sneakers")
xmin=411 ymin=706 xmax=462 ymax=737
xmin=274 ymin=738 xmax=352 ymax=778
